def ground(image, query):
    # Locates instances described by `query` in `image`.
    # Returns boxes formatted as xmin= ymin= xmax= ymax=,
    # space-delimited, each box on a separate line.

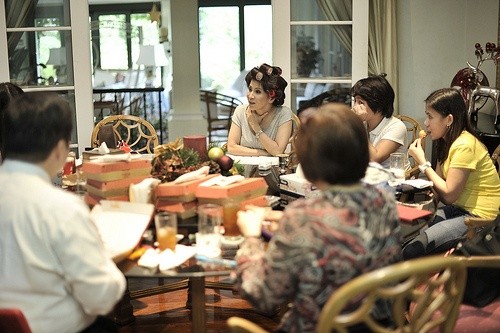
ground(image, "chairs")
xmin=394 ymin=114 xmax=425 ymax=181
xmin=227 ymin=256 xmax=466 ymax=333
xmin=90 ymin=115 xmax=158 ymax=156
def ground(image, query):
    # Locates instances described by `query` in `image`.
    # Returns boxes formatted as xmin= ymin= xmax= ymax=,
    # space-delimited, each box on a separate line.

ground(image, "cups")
xmin=61 ymin=153 xmax=79 ymax=175
xmin=391 ymin=153 xmax=407 ymax=182
xmin=154 ymin=212 xmax=177 ymax=254
xmin=196 ymin=204 xmax=223 ymax=258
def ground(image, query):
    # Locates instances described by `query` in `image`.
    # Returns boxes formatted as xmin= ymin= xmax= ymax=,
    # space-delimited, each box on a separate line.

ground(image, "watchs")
xmin=417 ymin=160 xmax=432 ymax=174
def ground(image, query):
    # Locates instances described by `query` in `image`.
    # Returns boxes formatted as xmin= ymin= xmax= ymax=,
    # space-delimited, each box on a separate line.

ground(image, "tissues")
xmin=196 ymin=172 xmax=270 ymax=199
xmin=82 ymin=140 xmax=156 ymax=204
xmin=156 ymin=166 xmax=222 ymax=196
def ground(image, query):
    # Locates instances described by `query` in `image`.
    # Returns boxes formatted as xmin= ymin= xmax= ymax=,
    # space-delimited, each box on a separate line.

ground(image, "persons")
xmin=0 ymin=80 xmax=126 ymax=333
xmin=401 ymin=88 xmax=499 ymax=261
xmin=227 ymin=63 xmax=294 ymax=156
xmin=347 ymin=72 xmax=407 ymax=170
xmin=232 ymin=103 xmax=405 ymax=333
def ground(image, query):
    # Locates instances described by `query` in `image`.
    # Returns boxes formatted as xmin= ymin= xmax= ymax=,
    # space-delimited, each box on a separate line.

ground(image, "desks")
xmin=112 ymin=262 xmax=243 ymax=333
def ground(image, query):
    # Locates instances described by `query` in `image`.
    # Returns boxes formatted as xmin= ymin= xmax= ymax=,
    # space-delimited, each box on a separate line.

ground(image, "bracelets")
xmin=255 ymin=128 xmax=263 ymax=138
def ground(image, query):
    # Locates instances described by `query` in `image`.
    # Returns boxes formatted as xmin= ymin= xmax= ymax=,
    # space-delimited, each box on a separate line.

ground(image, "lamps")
xmin=136 ymin=45 xmax=170 ymax=86
xmin=46 ymin=48 xmax=67 ymax=86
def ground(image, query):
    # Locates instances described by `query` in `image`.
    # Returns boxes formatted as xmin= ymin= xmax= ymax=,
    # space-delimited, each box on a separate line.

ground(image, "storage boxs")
xmin=81 ymin=159 xmax=268 ymax=236
